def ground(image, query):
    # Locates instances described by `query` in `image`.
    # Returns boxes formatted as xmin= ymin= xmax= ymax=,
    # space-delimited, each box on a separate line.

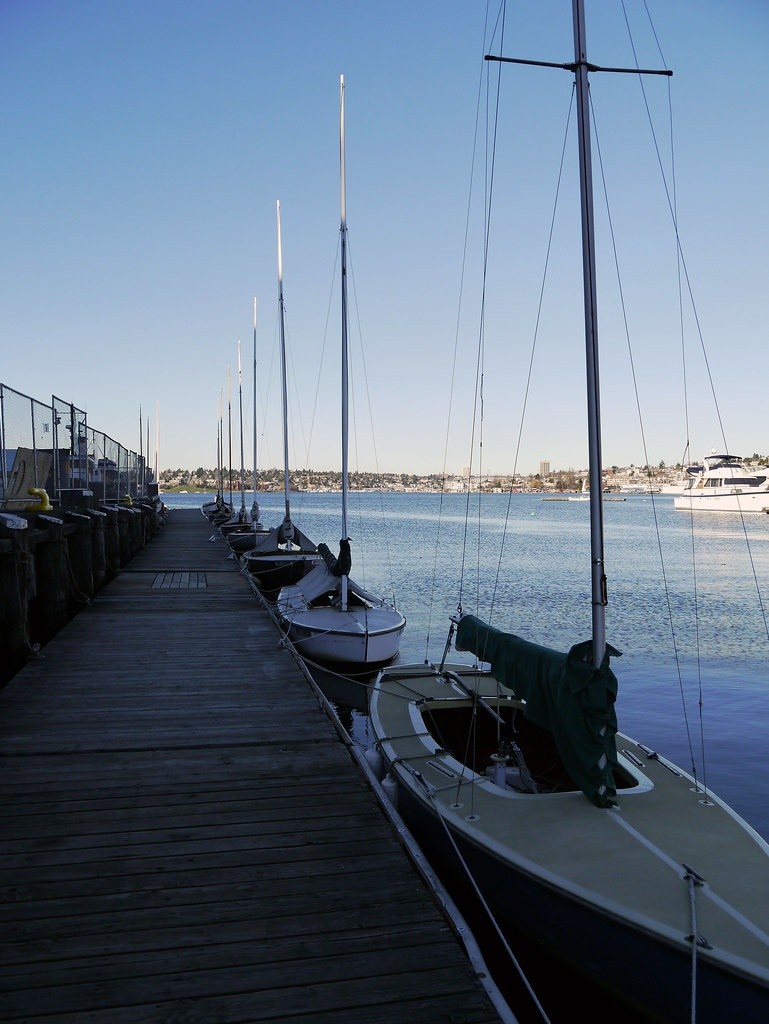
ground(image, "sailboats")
xmin=367 ymin=1 xmax=768 ymax=939
xmin=227 ymin=295 xmax=276 ymax=552
xmin=241 ymin=196 xmax=331 ymax=592
xmin=202 ymin=363 xmax=265 ymax=531
xmin=275 ymin=73 xmax=405 ymax=663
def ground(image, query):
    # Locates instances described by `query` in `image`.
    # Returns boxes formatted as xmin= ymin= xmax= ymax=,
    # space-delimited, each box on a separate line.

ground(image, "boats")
xmin=673 ymin=453 xmax=769 ymax=512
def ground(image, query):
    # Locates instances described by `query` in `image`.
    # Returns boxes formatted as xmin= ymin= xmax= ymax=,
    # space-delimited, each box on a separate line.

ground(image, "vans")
xmin=70 ymin=456 xmax=94 ymax=481
